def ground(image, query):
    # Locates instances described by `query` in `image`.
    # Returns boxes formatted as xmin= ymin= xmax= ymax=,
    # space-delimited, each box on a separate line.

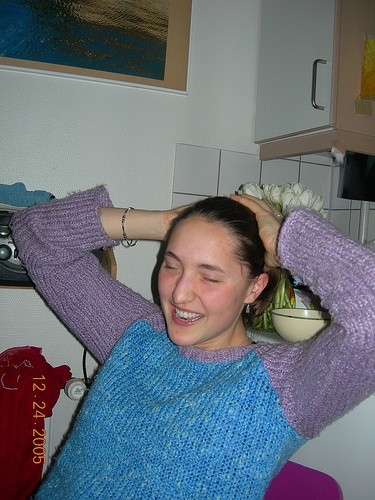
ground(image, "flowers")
xmin=237 ymin=181 xmax=329 ymax=329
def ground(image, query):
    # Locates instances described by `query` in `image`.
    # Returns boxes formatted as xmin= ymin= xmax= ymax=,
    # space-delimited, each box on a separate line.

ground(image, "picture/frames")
xmin=1 ymin=0 xmax=195 ymax=96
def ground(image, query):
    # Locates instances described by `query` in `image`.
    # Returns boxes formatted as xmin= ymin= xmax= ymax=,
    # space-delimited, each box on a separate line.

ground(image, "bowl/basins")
xmin=269 ymin=309 xmax=331 ymax=344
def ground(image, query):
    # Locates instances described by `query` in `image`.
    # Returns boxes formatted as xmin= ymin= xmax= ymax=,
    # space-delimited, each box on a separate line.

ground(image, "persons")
xmin=8 ymin=184 xmax=375 ymax=500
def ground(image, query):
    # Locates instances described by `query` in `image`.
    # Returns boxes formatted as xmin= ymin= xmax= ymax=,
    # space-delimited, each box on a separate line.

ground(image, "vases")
xmin=250 ymin=265 xmax=296 ymax=331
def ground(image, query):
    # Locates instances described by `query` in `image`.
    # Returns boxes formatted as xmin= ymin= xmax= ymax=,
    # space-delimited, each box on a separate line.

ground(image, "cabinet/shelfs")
xmin=254 ymin=0 xmax=374 ymax=161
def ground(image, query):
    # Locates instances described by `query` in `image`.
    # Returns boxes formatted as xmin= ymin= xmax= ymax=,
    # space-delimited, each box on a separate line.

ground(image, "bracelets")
xmin=122 ymin=207 xmax=138 ymax=247
